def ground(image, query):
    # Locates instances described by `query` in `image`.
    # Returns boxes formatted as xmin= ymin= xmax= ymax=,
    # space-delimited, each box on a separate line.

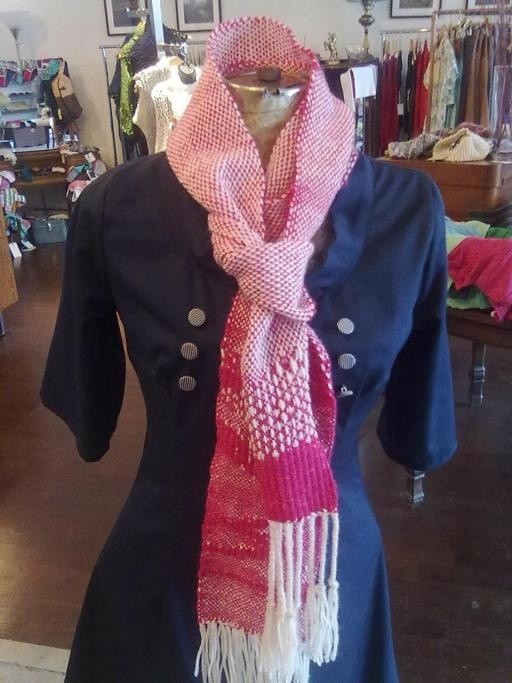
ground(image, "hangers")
xmin=157 ymin=41 xmax=193 ymax=64
xmin=376 ymin=6 xmax=511 ymax=67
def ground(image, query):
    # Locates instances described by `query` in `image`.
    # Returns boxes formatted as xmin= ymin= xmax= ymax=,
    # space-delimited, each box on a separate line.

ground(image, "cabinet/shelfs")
xmin=314 ymin=56 xmax=384 ymax=145
xmin=0 ymin=148 xmax=73 ymax=228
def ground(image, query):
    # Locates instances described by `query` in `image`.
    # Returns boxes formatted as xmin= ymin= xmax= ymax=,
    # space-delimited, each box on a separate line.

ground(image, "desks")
xmin=368 ymin=144 xmax=512 ymax=224
xmin=65 ymin=143 xmax=105 ymax=218
xmin=401 ymin=295 xmax=512 ymax=511
xmin=0 ymin=157 xmax=26 ymax=336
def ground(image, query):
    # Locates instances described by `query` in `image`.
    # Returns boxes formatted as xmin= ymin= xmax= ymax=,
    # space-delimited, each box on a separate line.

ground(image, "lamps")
xmin=1 ymin=7 xmax=33 ymax=78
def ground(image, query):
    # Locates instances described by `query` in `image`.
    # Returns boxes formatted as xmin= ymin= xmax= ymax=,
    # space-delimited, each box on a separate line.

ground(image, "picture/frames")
xmin=102 ymin=0 xmax=150 ymax=38
xmin=173 ymin=0 xmax=223 ymax=34
xmin=388 ymin=0 xmax=443 ymax=18
xmin=462 ymin=0 xmax=511 ymax=17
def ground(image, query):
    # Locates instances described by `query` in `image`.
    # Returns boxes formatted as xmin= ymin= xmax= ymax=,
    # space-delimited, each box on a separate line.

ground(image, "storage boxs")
xmin=6 ymin=119 xmax=51 ymax=148
xmin=31 ymin=215 xmax=70 ymax=245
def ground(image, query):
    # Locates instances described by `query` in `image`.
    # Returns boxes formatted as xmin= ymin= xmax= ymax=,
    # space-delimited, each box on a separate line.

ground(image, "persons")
xmin=39 ymin=14 xmax=461 ymax=683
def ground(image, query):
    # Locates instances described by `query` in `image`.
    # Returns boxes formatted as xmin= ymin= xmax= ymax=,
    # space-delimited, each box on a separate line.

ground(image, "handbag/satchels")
xmin=51 ymin=59 xmax=83 ymax=125
